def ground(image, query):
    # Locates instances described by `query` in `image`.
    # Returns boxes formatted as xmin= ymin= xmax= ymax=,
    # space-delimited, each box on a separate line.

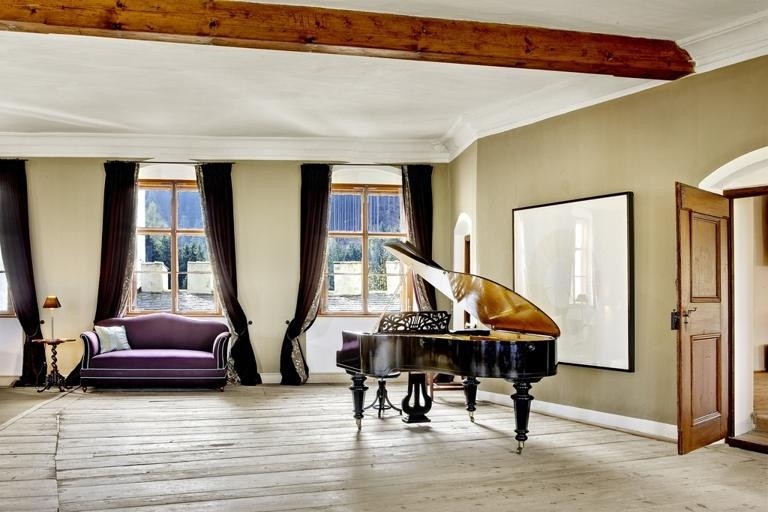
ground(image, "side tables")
xmin=32 ymin=338 xmax=75 ymax=393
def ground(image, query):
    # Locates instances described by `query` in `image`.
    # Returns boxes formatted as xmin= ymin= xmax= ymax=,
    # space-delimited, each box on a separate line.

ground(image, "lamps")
xmin=43 ymin=296 xmax=61 ymax=340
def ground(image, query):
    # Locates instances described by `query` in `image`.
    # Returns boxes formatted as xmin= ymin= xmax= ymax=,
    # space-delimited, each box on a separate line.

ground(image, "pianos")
xmin=336 ymin=239 xmax=561 ymax=453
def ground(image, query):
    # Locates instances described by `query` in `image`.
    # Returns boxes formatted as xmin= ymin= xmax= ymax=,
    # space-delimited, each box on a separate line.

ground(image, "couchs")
xmin=80 ymin=312 xmax=231 ymax=391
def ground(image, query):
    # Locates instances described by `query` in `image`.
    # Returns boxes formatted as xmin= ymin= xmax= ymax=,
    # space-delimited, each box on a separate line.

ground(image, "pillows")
xmin=94 ymin=324 xmax=131 ymax=354
